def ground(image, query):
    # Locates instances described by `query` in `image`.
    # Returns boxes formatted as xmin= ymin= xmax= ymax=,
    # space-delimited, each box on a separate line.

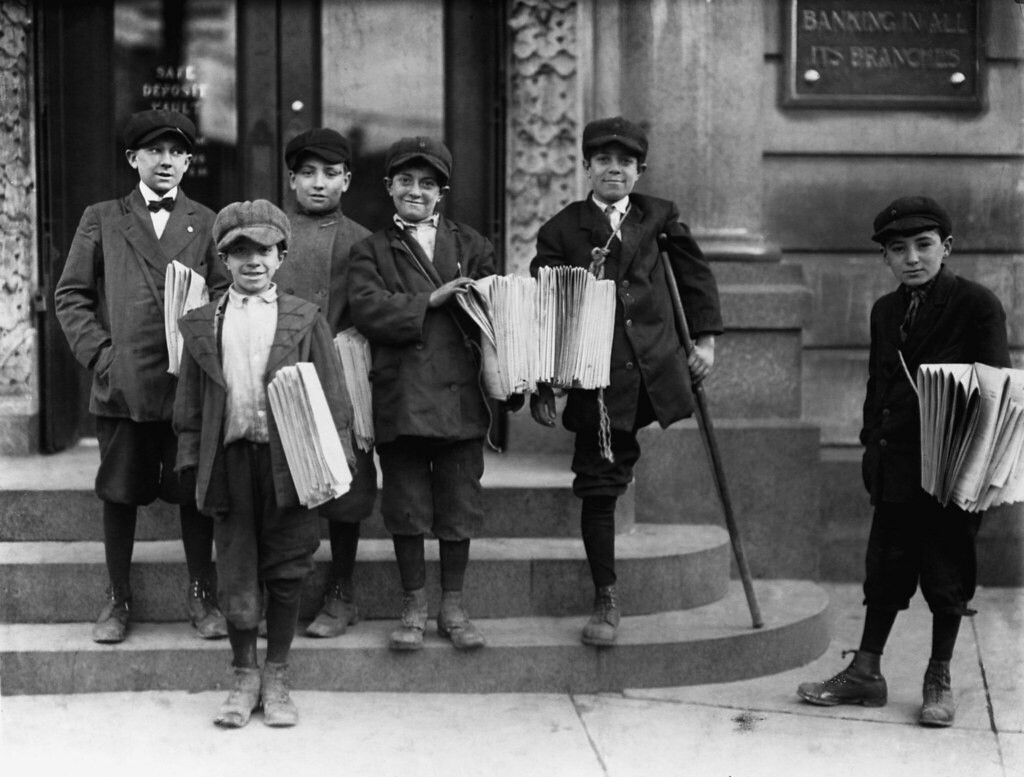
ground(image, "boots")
xmin=391 ymin=588 xmax=431 ymax=648
xmin=436 ymin=590 xmax=486 ymax=650
xmin=795 ymin=649 xmax=886 ymax=707
xmin=184 ymin=578 xmax=228 ymax=637
xmin=581 ymin=584 xmax=622 ymax=645
xmin=259 ymin=661 xmax=298 ymax=727
xmin=918 ymin=660 xmax=957 ymax=727
xmin=308 ymin=578 xmax=360 ymax=637
xmin=94 ymin=583 xmax=131 ymax=642
xmin=215 ymin=665 xmax=263 ymax=727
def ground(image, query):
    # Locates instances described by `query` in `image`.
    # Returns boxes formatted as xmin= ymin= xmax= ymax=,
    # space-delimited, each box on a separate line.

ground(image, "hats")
xmin=213 ymin=199 xmax=291 ymax=247
xmin=125 ymin=111 xmax=197 ymax=154
xmin=582 ymin=118 xmax=652 ymax=158
xmin=872 ymin=196 xmax=951 ymax=242
xmin=385 ymin=136 xmax=452 ymax=184
xmin=286 ymin=129 xmax=354 ymax=170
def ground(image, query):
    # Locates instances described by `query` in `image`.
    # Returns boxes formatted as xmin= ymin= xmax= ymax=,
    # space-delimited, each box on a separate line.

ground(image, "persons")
xmin=272 ymin=123 xmax=372 ymax=644
xmin=791 ymin=195 xmax=1013 ymax=729
xmin=51 ymin=105 xmax=235 ymax=639
xmin=529 ymin=109 xmax=721 ymax=649
xmin=174 ymin=200 xmax=365 ymax=727
xmin=346 ymin=133 xmax=531 ymax=645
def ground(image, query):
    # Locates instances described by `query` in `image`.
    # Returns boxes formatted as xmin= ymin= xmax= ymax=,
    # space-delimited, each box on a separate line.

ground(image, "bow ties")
xmin=147 ymin=196 xmax=175 ymax=212
